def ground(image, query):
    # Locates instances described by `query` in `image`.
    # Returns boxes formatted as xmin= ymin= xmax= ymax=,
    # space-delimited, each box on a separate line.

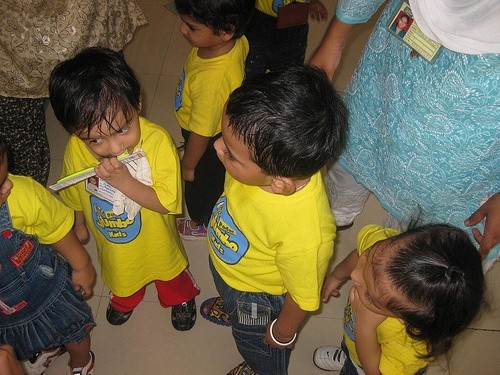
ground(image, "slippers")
xmin=226 ymin=361 xmax=259 ymax=375
xmin=201 ymin=296 xmax=231 ymax=326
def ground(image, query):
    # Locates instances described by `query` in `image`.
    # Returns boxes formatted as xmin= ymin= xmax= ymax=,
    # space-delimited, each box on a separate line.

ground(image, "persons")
xmin=308 ymin=0 xmax=500 ymax=275
xmin=0 ymin=343 xmax=24 ymax=375
xmin=49 ymin=46 xmax=200 ymax=331
xmin=312 ymin=200 xmax=484 ymax=375
xmin=245 ymin=0 xmax=327 ymax=81
xmin=391 ymin=14 xmax=409 ymax=38
xmin=171 ymin=0 xmax=249 ymax=241
xmin=0 ymin=0 xmax=149 ymax=187
xmin=0 ymin=142 xmax=96 ymax=375
xmin=201 ymin=64 xmax=350 ymax=375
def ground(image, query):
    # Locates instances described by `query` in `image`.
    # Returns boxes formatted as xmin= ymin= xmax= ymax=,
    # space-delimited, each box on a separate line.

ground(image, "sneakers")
xmin=26 ymin=344 xmax=95 ymax=375
xmin=171 ymin=297 xmax=196 ymax=331
xmin=106 ymin=303 xmax=134 ymax=326
xmin=314 ymin=345 xmax=347 ymax=371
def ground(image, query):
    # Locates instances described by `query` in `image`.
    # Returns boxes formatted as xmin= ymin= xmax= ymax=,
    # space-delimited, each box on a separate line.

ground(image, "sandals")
xmin=176 ymin=217 xmax=208 ymax=239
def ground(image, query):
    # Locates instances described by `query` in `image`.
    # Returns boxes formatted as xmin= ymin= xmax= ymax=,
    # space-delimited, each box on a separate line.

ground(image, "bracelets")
xmin=269 ymin=318 xmax=297 ymax=346
